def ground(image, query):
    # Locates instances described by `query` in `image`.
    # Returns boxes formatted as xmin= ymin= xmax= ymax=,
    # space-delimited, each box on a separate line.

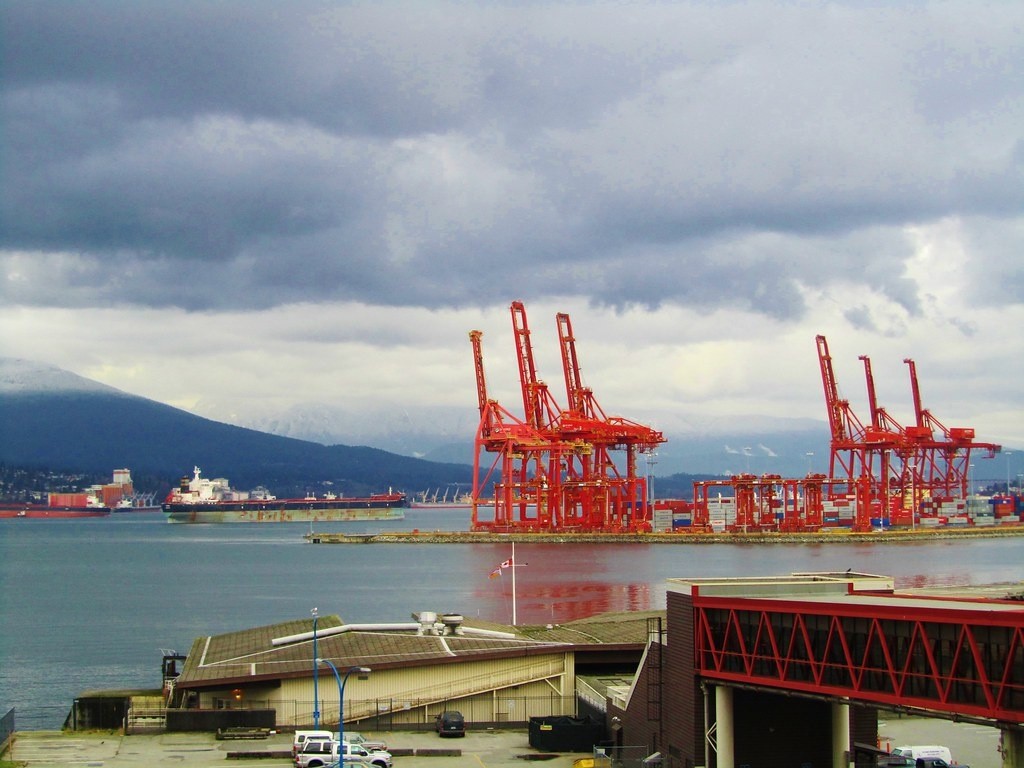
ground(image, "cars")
xmin=877 ymin=757 xmax=916 ymax=767
xmin=311 ymin=759 xmax=382 ymax=768
xmin=0 ymin=505 xmax=111 ymax=518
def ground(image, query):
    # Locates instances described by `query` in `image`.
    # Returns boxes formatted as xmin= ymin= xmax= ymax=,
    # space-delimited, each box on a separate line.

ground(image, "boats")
xmin=86 ymin=496 xmax=162 ymax=512
xmin=113 ymin=500 xmax=133 ymax=512
xmin=161 ymin=464 xmax=406 ymax=524
xmin=410 ymin=501 xmax=473 ymax=508
xmin=14 ymin=511 xmax=26 ymax=518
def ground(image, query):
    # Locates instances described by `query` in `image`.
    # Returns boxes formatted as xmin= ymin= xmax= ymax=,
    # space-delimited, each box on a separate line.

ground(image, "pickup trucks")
xmin=295 ymin=744 xmax=392 ymax=768
xmin=292 ymin=730 xmax=387 ymax=757
xmin=916 ymin=757 xmax=970 ymax=768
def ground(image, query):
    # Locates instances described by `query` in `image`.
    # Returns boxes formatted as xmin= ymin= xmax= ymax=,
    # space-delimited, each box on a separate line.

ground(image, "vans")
xmin=877 ymin=746 xmax=953 ymax=766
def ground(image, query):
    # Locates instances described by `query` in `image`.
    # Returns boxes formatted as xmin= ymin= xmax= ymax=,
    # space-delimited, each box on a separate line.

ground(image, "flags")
xmin=488 ymin=569 xmax=500 ymax=579
xmin=500 ymin=559 xmax=512 ymax=568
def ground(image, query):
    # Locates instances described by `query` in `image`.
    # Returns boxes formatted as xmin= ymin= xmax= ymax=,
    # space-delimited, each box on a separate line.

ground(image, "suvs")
xmin=435 ymin=710 xmax=466 ymax=737
xmin=302 ymin=738 xmax=364 ymax=752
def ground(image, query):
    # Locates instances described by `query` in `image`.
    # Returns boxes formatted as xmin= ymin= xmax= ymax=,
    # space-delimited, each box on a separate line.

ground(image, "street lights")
xmin=908 ymin=465 xmax=918 ymax=529
xmin=969 ymin=465 xmax=976 ymax=524
xmin=1005 ymin=452 xmax=1012 ymax=495
xmin=315 ymin=658 xmax=372 ymax=768
xmin=805 ymin=452 xmax=814 ymax=476
xmin=1017 ymin=473 xmax=1022 ymax=495
xmin=310 ymin=607 xmax=320 ymax=731
xmin=648 ymin=452 xmax=657 ymax=532
